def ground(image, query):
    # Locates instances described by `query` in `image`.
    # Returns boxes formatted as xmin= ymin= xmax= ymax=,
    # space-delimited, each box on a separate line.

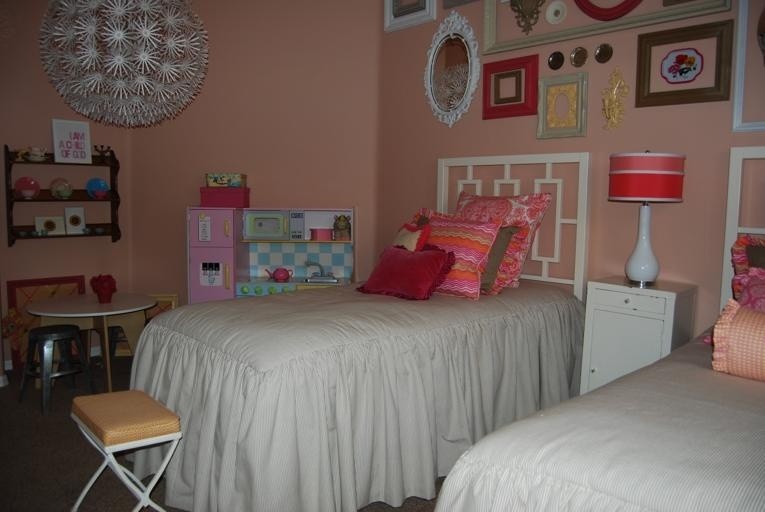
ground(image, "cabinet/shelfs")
xmin=183 ymin=203 xmax=356 ymax=304
xmin=2 ymin=143 xmax=124 ymax=248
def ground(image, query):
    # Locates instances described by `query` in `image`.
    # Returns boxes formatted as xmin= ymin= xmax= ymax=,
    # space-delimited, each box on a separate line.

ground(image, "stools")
xmin=68 ymin=388 xmax=183 ymax=512
xmin=17 ymin=324 xmax=98 ymax=416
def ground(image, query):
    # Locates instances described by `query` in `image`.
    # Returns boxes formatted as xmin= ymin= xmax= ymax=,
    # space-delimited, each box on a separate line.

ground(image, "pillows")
xmin=702 ymin=234 xmax=765 ymax=383
xmin=356 ymin=189 xmax=553 ymax=302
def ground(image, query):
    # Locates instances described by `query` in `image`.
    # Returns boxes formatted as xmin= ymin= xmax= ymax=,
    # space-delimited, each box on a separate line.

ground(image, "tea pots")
xmin=264 ymin=267 xmax=295 ymax=282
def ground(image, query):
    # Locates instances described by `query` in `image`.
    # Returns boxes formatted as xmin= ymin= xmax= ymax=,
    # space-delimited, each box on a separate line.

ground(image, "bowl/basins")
xmin=57 ymin=190 xmax=74 ymax=200
xmin=93 ymin=190 xmax=109 ymax=200
xmin=21 ymin=190 xmax=36 ymax=201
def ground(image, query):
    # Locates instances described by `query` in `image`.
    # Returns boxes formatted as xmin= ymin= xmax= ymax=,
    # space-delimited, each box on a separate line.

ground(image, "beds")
xmin=129 ymin=148 xmax=592 ymax=512
xmin=429 ymin=144 xmax=765 ymax=511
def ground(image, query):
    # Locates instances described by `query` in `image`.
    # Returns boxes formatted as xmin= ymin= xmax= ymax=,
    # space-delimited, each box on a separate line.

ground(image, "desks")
xmin=23 ymin=292 xmax=158 ymax=392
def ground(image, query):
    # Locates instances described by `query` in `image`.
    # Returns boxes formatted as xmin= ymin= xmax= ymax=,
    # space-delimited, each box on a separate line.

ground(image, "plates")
xmin=86 ymin=178 xmax=110 ymax=199
xmin=15 ymin=176 xmax=41 ymax=199
xmin=24 ymin=155 xmax=48 ymax=161
xmin=49 ymin=177 xmax=74 ymax=199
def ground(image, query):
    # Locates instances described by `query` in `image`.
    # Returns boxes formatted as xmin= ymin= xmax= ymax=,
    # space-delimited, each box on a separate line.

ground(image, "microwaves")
xmin=243 ymin=211 xmax=290 ymax=242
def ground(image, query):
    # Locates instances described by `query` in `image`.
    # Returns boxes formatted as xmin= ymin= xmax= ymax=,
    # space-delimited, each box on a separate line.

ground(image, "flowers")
xmin=1 ymin=307 xmax=31 ymax=352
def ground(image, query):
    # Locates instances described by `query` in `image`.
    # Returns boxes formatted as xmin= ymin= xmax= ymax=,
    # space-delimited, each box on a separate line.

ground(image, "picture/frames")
xmin=633 ymin=19 xmax=736 ymax=110
xmin=113 ymin=293 xmax=178 ymax=357
xmin=535 ymin=70 xmax=589 ymax=140
xmin=381 ymin=0 xmax=437 ymax=35
xmin=6 ymin=274 xmax=87 ymax=372
xmin=481 ymin=54 xmax=541 ymax=121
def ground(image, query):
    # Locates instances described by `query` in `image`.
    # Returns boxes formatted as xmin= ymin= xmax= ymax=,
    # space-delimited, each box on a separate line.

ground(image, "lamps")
xmin=606 ymin=148 xmax=688 ymax=288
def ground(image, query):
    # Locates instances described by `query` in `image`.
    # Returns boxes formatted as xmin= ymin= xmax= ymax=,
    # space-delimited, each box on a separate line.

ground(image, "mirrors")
xmin=420 ymin=8 xmax=482 ymax=127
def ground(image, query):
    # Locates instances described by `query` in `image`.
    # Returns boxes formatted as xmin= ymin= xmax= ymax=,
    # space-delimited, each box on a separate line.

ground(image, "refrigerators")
xmin=186 ymin=208 xmax=250 ymax=306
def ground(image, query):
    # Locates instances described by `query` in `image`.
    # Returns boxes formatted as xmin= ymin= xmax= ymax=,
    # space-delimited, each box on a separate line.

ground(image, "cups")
xmin=95 ymin=279 xmax=115 ymax=304
xmin=29 ymin=145 xmax=48 ymax=157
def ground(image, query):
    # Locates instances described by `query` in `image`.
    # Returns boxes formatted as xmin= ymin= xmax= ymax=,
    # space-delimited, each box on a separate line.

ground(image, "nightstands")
xmin=579 ymin=273 xmax=699 ymax=398
